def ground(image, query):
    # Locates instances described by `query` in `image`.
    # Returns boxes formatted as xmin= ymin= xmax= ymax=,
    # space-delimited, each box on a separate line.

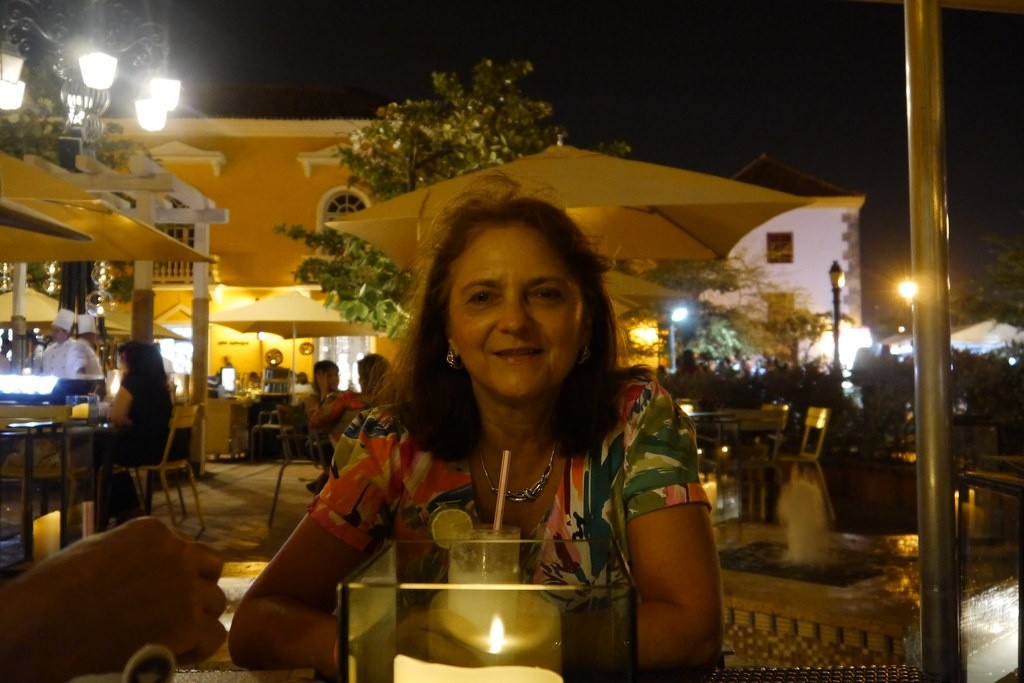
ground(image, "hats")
xmin=52 ymin=308 xmax=76 ymax=334
xmin=76 ymin=314 xmax=98 ymax=334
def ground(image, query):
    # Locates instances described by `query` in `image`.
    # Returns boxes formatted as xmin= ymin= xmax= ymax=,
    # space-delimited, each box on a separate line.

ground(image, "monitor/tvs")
xmin=221 ymin=367 xmax=236 ymax=393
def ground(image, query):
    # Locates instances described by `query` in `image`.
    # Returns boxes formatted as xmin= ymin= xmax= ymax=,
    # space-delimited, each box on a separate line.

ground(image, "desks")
xmin=709 ymin=408 xmax=787 ymax=434
xmin=0 ymin=419 xmax=112 ymax=558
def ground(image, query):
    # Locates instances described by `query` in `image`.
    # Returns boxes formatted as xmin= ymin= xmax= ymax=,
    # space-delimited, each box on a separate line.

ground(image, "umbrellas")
xmin=209 ymin=290 xmax=375 ymax=402
xmin=602 ymin=271 xmax=680 ymax=306
xmin=325 ymin=134 xmax=816 ymax=264
xmin=0 ymin=151 xmax=217 ymax=264
xmin=0 ymin=287 xmax=184 ymax=339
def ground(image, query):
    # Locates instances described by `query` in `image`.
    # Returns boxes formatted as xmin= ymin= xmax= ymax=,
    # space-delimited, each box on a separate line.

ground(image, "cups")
xmin=445 ymin=523 xmax=521 ymax=619
xmin=87 ymin=394 xmax=99 ymax=427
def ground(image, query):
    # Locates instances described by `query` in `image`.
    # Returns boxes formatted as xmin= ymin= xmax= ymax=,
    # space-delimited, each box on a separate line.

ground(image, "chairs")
xmin=684 ymin=401 xmax=831 ymax=522
xmin=98 ymin=403 xmax=207 ymax=532
xmin=251 ymin=405 xmax=329 ymax=528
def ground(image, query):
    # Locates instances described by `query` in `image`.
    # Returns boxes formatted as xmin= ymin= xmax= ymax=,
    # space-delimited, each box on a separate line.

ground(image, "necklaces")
xmin=478 ymin=442 xmax=557 ymax=502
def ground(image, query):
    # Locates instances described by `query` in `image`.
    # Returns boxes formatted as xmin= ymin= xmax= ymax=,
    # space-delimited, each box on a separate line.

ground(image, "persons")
xmin=658 ymin=350 xmax=821 ymax=410
xmin=0 ymin=517 xmax=228 ymax=683
xmin=228 ymin=197 xmax=723 ymax=681
xmin=303 ymin=354 xmax=393 ymax=497
xmin=95 ymin=341 xmax=173 ymax=530
xmin=41 ymin=309 xmax=102 ymax=375
